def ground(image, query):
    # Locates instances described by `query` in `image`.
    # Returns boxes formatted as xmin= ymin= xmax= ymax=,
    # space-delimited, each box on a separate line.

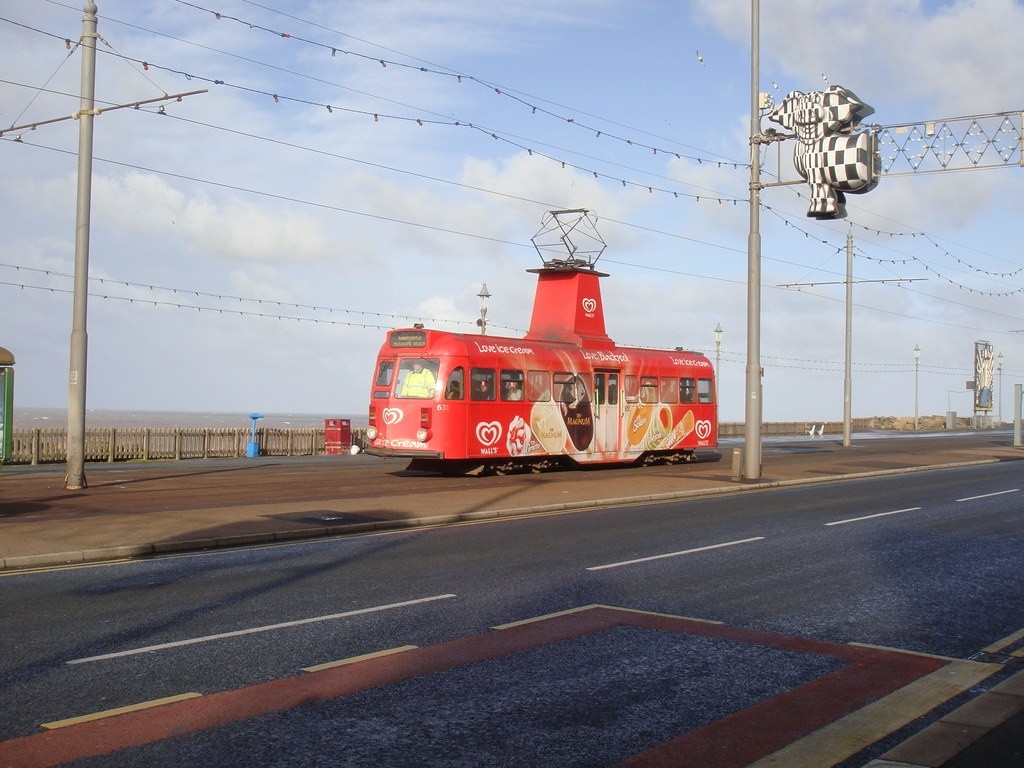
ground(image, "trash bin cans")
xmin=325 ymin=418 xmax=351 ymax=456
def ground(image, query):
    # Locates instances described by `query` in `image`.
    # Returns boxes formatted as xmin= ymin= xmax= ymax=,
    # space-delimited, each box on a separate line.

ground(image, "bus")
xmin=365 ymin=207 xmax=721 ymax=475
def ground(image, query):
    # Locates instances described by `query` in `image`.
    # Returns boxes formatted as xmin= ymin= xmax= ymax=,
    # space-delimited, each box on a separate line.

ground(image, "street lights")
xmin=478 ymin=282 xmax=493 ymax=334
xmin=998 ymin=351 xmax=1003 ymax=423
xmin=713 ymin=322 xmax=724 ymax=419
xmin=911 ymin=343 xmax=921 ymax=429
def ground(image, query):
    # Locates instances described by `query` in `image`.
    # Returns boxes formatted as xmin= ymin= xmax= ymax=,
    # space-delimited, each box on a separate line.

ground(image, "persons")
xmin=506 ymin=381 xmax=522 ymax=400
xmin=448 ymin=381 xmax=460 ymax=399
xmin=401 ymin=363 xmax=436 ymax=398
xmin=474 ymin=381 xmax=493 ymax=400
xmin=687 ymin=388 xmax=701 ymax=402
